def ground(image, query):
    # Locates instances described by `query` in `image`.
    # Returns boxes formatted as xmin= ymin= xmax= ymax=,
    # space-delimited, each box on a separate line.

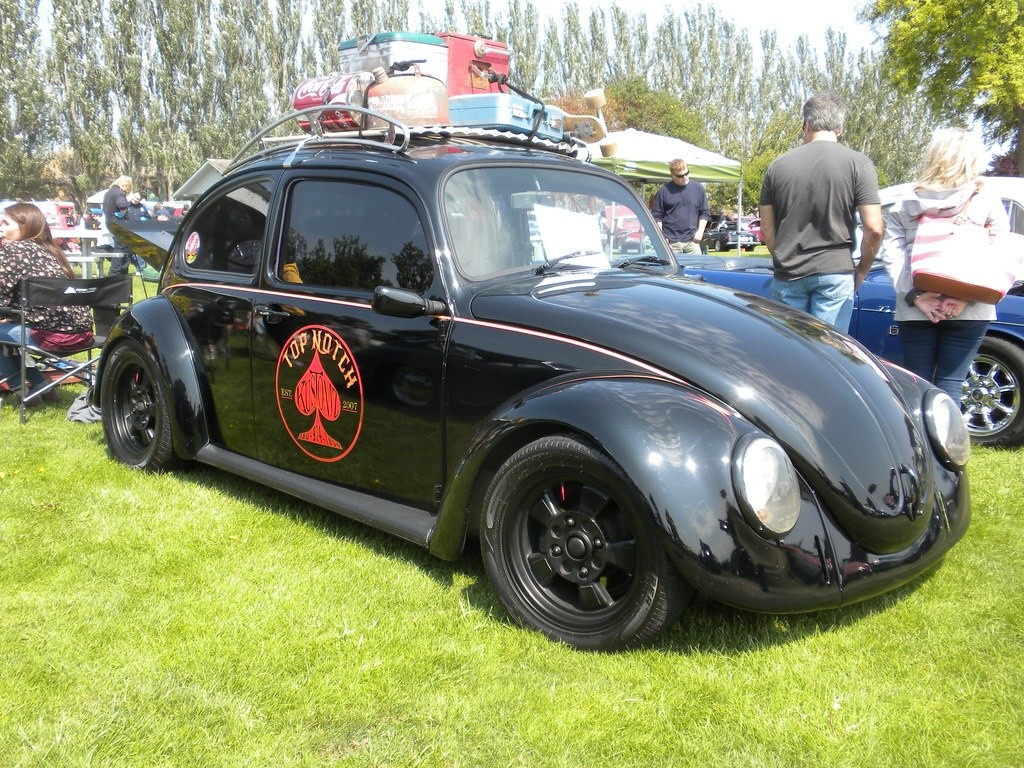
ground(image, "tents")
xmin=590 ymin=127 xmax=743 ymax=261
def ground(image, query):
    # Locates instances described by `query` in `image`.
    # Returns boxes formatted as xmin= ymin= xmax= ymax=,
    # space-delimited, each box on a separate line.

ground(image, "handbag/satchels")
xmin=908 ymin=183 xmax=1024 ymax=305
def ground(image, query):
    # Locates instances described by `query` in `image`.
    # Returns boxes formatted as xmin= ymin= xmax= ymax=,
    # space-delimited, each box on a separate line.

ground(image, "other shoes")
xmin=19 ymin=392 xmax=41 ymax=406
xmin=38 ymin=387 xmax=62 ymax=403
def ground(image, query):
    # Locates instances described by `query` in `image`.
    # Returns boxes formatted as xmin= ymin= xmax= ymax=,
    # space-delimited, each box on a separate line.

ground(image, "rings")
xmin=951 ymin=316 xmax=952 ymax=317
xmin=932 ymin=311 xmax=935 ymax=314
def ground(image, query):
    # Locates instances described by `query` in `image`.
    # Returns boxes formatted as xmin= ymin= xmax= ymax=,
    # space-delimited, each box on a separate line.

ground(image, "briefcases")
xmin=448 ymin=95 xmax=565 ymax=141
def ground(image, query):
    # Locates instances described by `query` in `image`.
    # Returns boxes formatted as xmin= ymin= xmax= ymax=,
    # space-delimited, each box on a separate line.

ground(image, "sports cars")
xmin=606 ymin=254 xmax=1024 ymax=452
xmin=704 ymin=222 xmax=761 ymax=252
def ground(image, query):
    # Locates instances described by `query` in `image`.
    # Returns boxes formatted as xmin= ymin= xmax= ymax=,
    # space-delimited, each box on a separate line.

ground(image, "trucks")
xmin=0 ymin=202 xmax=79 ymax=252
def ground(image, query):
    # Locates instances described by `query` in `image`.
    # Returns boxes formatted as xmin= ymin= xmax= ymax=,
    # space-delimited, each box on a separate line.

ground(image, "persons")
xmin=102 ymin=176 xmax=133 ymax=276
xmin=127 ymin=192 xmax=150 ymax=275
xmin=760 ymin=91 xmax=883 ymax=334
xmin=653 ymin=159 xmax=709 ymax=254
xmin=153 ymin=201 xmax=189 ymax=223
xmin=71 ymin=209 xmax=114 ymax=277
xmin=882 ymin=128 xmax=1008 ymax=406
xmin=0 ymin=203 xmax=93 ymax=406
xmin=719 ymin=210 xmax=734 ymax=225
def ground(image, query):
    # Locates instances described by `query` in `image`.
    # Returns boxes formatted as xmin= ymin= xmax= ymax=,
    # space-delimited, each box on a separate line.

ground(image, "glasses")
xmin=672 ymin=171 xmax=690 ymax=178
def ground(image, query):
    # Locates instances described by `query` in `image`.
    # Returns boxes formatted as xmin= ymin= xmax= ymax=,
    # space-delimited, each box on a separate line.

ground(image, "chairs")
xmin=0 ymin=273 xmax=133 ymax=424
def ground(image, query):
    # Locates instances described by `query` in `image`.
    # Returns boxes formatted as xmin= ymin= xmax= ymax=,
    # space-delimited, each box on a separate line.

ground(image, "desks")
xmin=51 ymin=230 xmax=115 ymax=279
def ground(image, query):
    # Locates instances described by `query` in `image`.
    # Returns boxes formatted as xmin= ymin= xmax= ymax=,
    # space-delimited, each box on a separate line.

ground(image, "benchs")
xmin=64 ymin=252 xmax=125 ymax=278
xmin=66 ymin=257 xmax=101 ymax=278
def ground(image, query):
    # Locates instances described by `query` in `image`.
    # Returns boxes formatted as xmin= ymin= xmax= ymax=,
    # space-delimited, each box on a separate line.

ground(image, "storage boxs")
xmin=338 ymin=32 xmax=449 ymax=84
xmin=435 ymin=32 xmax=510 ymax=98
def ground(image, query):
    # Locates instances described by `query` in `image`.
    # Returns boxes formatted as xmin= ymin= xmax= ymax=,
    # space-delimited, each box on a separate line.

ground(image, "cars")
xmin=734 ymin=217 xmax=765 ymax=243
xmin=527 ymin=213 xmax=542 ymax=245
xmin=854 ymin=177 xmax=1024 ymax=285
xmin=596 ymin=205 xmax=653 ymax=252
xmin=86 ymin=102 xmax=971 ymax=652
